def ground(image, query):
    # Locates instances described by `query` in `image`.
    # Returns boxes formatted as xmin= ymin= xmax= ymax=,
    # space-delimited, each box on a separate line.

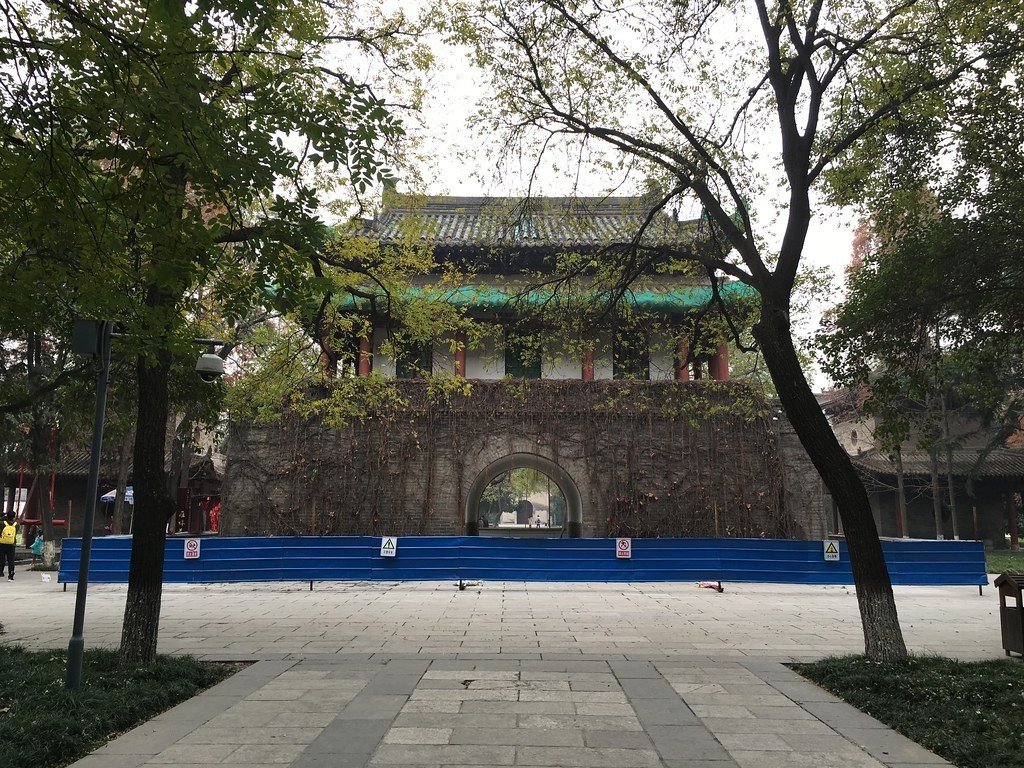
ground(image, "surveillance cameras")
xmin=195 ymin=353 xmax=225 ymax=383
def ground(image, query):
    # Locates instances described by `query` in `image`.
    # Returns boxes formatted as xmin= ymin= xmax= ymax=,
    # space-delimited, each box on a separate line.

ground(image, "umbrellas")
xmin=100 ymin=485 xmax=135 ymax=535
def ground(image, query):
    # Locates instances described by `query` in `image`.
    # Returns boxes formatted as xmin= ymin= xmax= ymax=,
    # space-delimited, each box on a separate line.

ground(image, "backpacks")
xmin=0 ymin=521 xmax=17 ymax=544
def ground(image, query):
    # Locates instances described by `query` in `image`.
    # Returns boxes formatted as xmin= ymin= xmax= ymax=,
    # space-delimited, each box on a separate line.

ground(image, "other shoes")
xmin=8 ymin=577 xmax=15 ymax=582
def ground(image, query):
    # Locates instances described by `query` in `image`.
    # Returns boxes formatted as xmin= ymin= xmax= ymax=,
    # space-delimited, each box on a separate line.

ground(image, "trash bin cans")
xmin=993 ymin=571 xmax=1024 ymax=656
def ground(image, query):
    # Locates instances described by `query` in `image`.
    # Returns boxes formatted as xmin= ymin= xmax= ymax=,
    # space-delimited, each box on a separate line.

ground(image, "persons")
xmin=0 ymin=510 xmax=20 ymax=581
xmin=535 ymin=515 xmax=541 ymax=528
xmin=29 ymin=529 xmax=44 ymax=565
xmin=478 ymin=516 xmax=489 ymax=528
xmin=528 ymin=516 xmax=533 ymax=528
xmin=105 ymin=514 xmax=114 ymax=535
